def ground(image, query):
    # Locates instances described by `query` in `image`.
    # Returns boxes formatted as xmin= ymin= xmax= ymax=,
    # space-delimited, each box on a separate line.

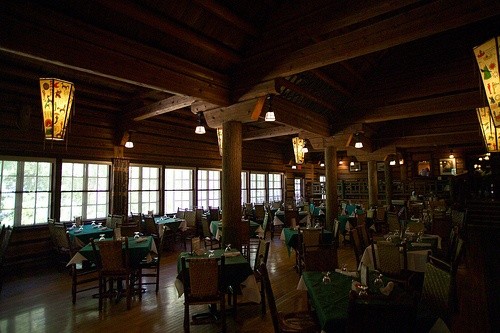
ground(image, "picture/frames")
xmin=376 ymin=162 xmax=386 ymax=172
xmin=348 ymin=161 xmax=362 ymax=172
xmin=439 ymin=159 xmax=456 ymax=176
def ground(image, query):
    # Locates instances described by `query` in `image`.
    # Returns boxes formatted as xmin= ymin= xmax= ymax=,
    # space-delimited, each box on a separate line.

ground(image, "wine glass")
xmin=314 ymin=222 xmax=320 ymax=229
xmin=189 ymin=243 xmax=233 ymax=259
xmin=133 ymin=231 xmax=140 ymax=240
xmin=160 ymin=212 xmax=176 ymax=221
xmin=72 ymin=220 xmax=103 ymax=231
xmin=321 ymin=263 xmax=386 ymax=298
xmin=386 ymin=228 xmax=424 ymax=243
xmin=98 ymin=234 xmax=106 ymax=241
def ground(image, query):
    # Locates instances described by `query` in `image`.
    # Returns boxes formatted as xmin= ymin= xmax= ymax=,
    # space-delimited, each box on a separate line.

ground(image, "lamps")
xmin=265 ymin=96 xmax=276 ymax=122
xmin=196 ymin=114 xmax=205 ymax=134
xmin=125 ymin=133 xmax=133 ymax=148
xmin=39 ymin=76 xmax=76 ymax=151
xmin=355 ymin=134 xmax=363 ymax=148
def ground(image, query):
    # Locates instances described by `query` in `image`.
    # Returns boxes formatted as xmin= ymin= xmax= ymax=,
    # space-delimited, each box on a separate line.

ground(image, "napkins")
xmin=335 ymin=269 xmax=360 ymax=278
xmin=223 ymin=252 xmax=241 ymax=256
xmin=100 ymin=227 xmax=107 ymax=230
xmin=137 ymin=237 xmax=147 ymax=243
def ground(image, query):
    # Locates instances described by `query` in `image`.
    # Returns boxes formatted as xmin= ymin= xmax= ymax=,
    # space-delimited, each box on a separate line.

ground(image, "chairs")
xmin=2 ymin=191 xmax=465 ymax=333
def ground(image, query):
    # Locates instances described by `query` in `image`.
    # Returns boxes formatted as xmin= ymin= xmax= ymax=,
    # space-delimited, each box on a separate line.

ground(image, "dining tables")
xmin=67 ymin=235 xmax=157 ymax=302
xmin=153 ymin=216 xmax=186 ymax=251
xmin=176 ymin=249 xmax=252 ymax=311
xmin=338 ymin=215 xmax=373 ymax=240
xmin=274 ymin=211 xmax=307 ymax=228
xmin=208 ymin=219 xmax=260 ymax=248
xmin=358 ymin=232 xmax=442 ymax=269
xmin=279 ymin=228 xmax=334 ymax=258
xmin=64 ymin=224 xmax=113 ymax=250
xmin=300 ymin=270 xmax=367 ymax=332
